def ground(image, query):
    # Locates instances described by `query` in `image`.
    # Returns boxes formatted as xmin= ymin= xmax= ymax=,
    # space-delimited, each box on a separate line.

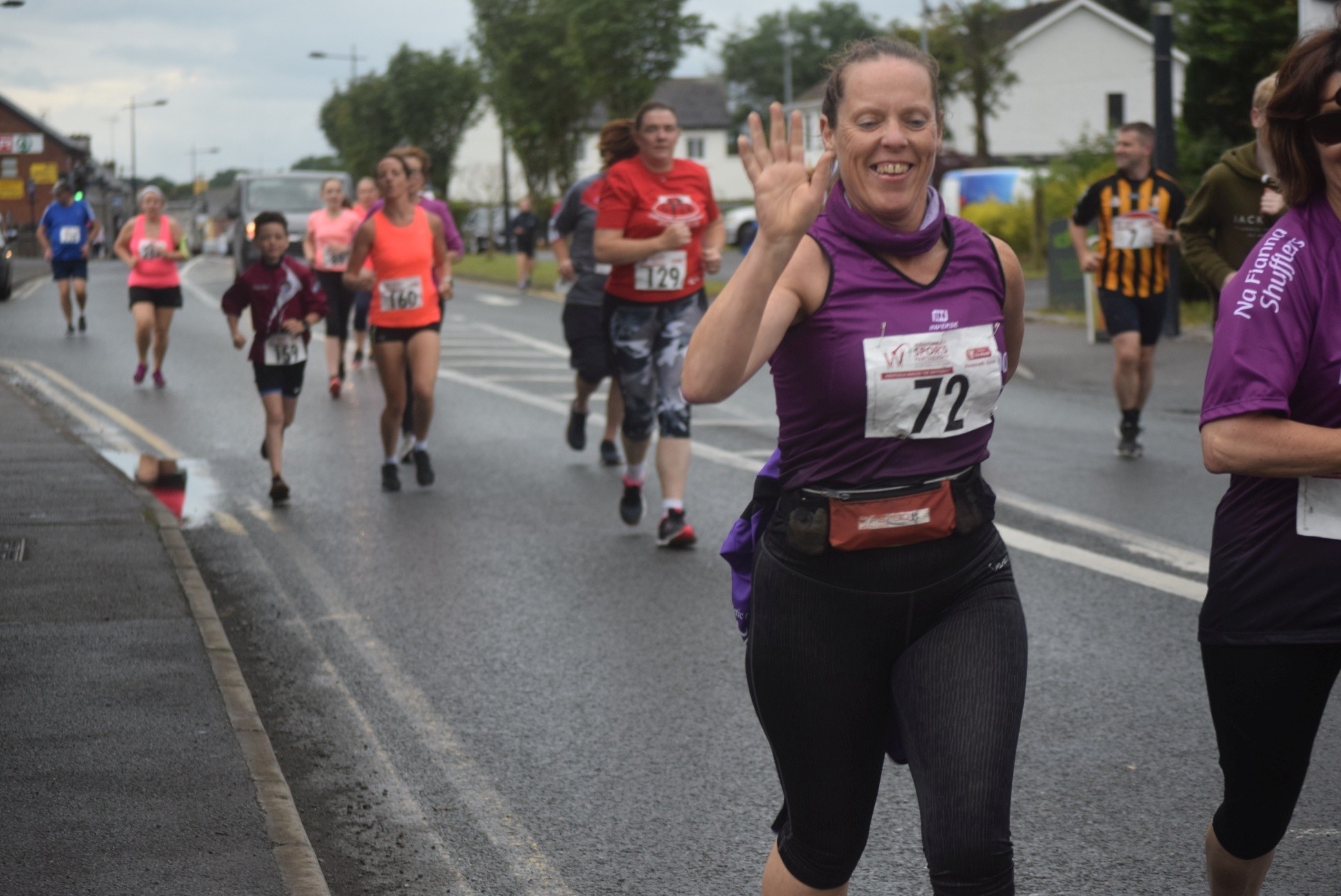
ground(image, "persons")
xmin=551 ymin=122 xmax=639 ymax=466
xmin=115 ymin=185 xmax=190 ymax=386
xmin=1201 ymin=1 xmax=1341 ymax=895
xmin=676 ymin=35 xmax=1031 ymax=896
xmin=39 ymin=180 xmax=99 ymax=338
xmin=1072 ymin=120 xmax=1181 ymax=459
xmin=308 ymin=148 xmax=463 ymax=491
xmin=510 ymin=196 xmax=538 ymax=289
xmin=1181 ymin=72 xmax=1285 ymax=336
xmin=584 ymin=104 xmax=724 ymax=548
xmin=222 ymin=211 xmax=327 ymax=503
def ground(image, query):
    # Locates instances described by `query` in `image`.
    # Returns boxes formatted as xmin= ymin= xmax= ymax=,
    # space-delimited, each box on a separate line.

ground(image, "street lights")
xmin=125 ymin=89 xmax=168 ymax=225
xmin=310 ymin=44 xmax=361 ymax=198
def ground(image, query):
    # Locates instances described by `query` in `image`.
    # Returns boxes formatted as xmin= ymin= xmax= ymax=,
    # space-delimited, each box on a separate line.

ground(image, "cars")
xmin=723 ymin=204 xmax=757 ymax=254
xmin=462 ymin=205 xmax=525 ymax=255
xmin=0 ymin=213 xmax=18 ymax=302
xmin=163 ymin=201 xmax=209 ymax=255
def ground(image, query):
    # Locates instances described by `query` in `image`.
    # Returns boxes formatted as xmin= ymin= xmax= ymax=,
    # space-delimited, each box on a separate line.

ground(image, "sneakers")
xmin=620 ymin=474 xmax=645 ymax=527
xmin=656 ymin=507 xmax=696 ymax=546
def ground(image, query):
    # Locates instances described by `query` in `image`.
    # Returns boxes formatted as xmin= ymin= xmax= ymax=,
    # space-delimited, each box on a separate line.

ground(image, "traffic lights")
xmin=72 ymin=169 xmax=87 ymax=204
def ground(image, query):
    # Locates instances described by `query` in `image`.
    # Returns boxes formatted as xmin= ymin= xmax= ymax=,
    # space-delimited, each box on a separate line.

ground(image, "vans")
xmin=222 ymin=170 xmax=353 ymax=290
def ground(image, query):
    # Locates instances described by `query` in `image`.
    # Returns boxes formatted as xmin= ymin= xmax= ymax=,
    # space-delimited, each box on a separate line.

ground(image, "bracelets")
xmin=1169 ymin=231 xmax=1176 ymax=243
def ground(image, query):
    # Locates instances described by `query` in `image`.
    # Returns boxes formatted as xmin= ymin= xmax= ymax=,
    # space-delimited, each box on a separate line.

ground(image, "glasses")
xmin=1310 ymin=109 xmax=1340 ymax=146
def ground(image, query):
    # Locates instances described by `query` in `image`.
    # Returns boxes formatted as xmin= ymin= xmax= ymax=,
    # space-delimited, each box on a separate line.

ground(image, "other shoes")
xmin=400 ymin=436 xmax=416 ymax=462
xmin=66 ymin=325 xmax=74 ymax=338
xmin=381 ymin=462 xmax=402 ymax=492
xmin=601 ymin=440 xmax=622 ymax=466
xmin=260 ymin=428 xmax=284 ymax=459
xmin=1115 ymin=428 xmax=1142 ymax=458
xmin=133 ymin=364 xmax=147 ymax=384
xmin=411 ymin=445 xmax=435 ymax=486
xmin=268 ymin=476 xmax=291 ymax=503
xmin=331 ymin=378 xmax=339 ymax=399
xmin=567 ymin=399 xmax=589 ymax=450
xmin=78 ymin=315 xmax=86 ymax=330
xmin=152 ymin=369 xmax=165 ymax=387
